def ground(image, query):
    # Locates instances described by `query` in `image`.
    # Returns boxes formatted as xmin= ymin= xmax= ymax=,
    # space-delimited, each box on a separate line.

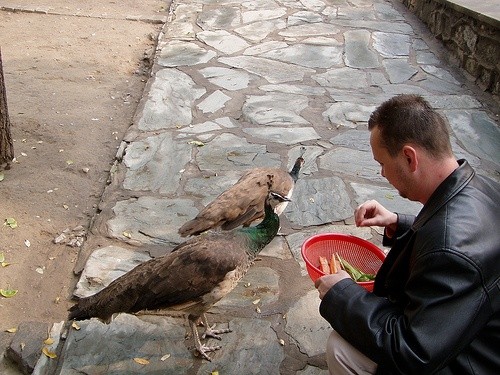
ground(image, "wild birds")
xmin=66 ymin=174 xmax=292 ymax=363
xmin=177 ymin=147 xmax=307 ymax=265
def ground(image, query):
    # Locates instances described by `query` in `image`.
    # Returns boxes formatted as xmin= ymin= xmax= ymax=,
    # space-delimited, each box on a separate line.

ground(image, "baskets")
xmin=301 ymin=232 xmax=387 ymax=294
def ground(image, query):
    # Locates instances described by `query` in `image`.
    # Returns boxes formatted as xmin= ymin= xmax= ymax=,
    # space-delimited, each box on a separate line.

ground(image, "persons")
xmin=315 ymin=93 xmax=500 ymax=374
